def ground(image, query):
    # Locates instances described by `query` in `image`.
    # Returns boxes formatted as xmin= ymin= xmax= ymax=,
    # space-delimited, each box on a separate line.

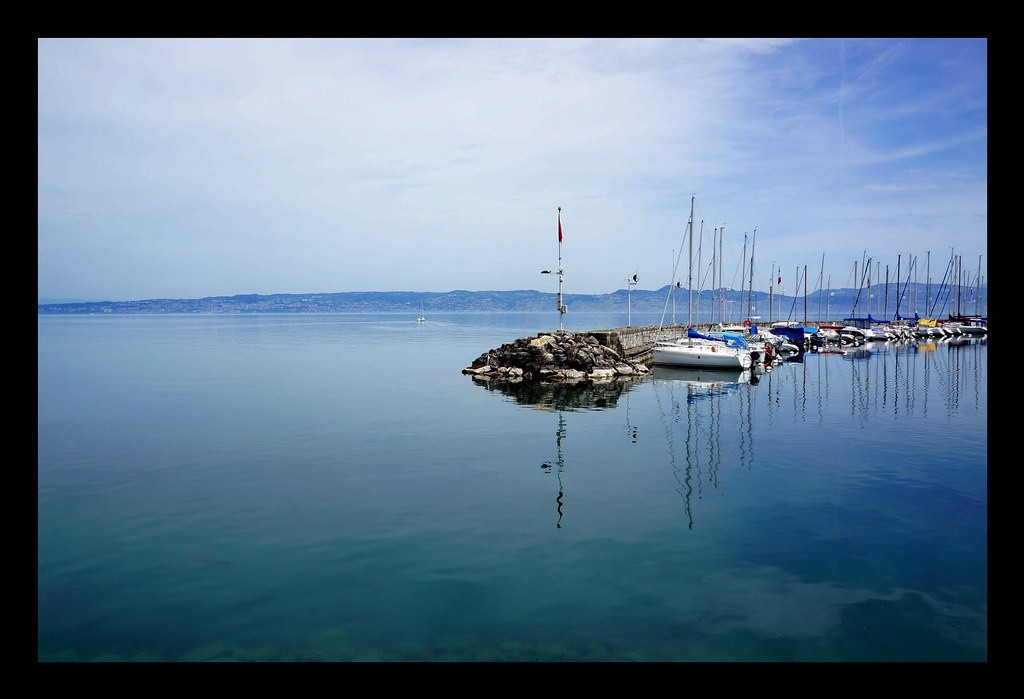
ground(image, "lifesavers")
xmin=743 ymin=320 xmax=751 ymax=327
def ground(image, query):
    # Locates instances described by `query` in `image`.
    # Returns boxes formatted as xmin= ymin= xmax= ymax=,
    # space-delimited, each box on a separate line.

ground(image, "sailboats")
xmin=417 ymin=296 xmax=425 ymax=322
xmin=651 ymin=193 xmax=988 ymax=372
xmin=652 ymin=366 xmax=751 ymax=530
